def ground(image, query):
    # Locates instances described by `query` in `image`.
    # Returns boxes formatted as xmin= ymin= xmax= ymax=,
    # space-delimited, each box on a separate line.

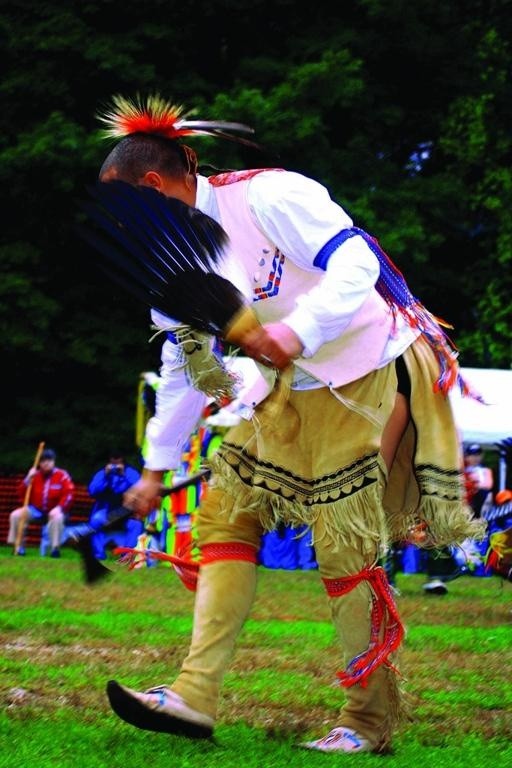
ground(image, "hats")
xmin=40 ymin=449 xmax=56 ymax=461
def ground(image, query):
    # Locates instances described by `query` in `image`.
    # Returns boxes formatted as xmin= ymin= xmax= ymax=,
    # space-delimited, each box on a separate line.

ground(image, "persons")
xmin=99 ymin=94 xmax=462 ymax=756
xmin=87 ymin=455 xmax=144 ymax=560
xmin=386 ymin=541 xmax=449 ymax=596
xmin=7 ymin=451 xmax=75 ymax=557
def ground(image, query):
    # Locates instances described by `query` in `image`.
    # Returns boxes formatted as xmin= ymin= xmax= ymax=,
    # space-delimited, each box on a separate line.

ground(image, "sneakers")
xmin=423 ymin=582 xmax=449 ymax=595
xmin=291 ymin=727 xmax=373 ymax=755
xmin=52 ymin=548 xmax=60 ymax=558
xmin=107 ymin=680 xmax=214 ymax=739
xmin=18 ymin=547 xmax=25 ymax=556
